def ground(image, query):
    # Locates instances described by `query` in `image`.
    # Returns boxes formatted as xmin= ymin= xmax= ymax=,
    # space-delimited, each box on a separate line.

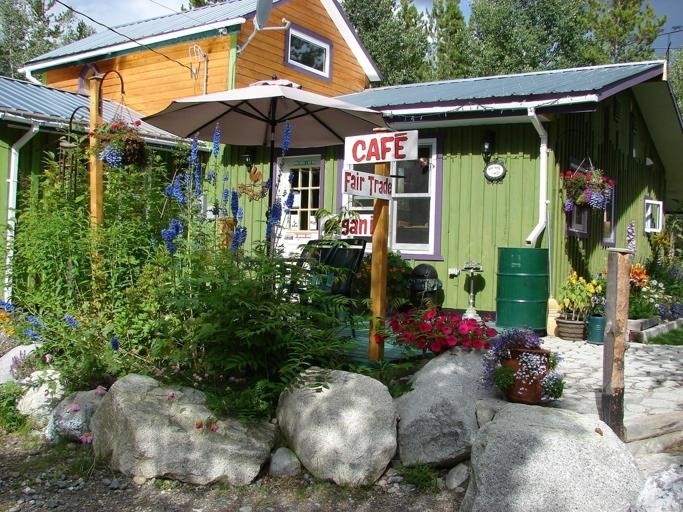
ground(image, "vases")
xmin=587 ymin=317 xmax=605 ymax=342
xmin=501 ymin=348 xmax=549 ymax=405
xmin=555 ymin=318 xmax=585 ymax=341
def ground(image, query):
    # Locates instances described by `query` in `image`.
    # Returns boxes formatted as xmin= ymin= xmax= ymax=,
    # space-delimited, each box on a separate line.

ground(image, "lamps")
xmin=240 ymin=147 xmax=255 ymax=172
xmin=480 ymin=134 xmax=495 ymax=165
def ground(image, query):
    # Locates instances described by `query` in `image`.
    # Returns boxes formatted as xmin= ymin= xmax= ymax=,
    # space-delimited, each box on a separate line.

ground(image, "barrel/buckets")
xmin=496 ymin=246 xmax=547 ymax=329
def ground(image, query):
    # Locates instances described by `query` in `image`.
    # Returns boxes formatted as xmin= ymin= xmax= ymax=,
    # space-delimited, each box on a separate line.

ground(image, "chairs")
xmin=286 ymin=238 xmax=366 ymax=338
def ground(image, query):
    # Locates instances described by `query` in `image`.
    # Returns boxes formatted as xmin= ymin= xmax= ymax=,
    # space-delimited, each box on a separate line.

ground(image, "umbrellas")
xmin=139 ymin=73 xmax=393 ymax=258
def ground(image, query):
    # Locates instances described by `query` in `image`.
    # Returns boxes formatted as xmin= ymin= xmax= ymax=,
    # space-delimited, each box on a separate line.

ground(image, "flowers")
xmin=480 ymin=326 xmax=566 ymax=400
xmin=89 ymin=120 xmax=150 ymax=170
xmin=559 ymin=167 xmax=616 ymax=212
xmin=556 ymin=271 xmax=602 ymax=321
xmin=591 ymin=296 xmax=607 ymax=317
